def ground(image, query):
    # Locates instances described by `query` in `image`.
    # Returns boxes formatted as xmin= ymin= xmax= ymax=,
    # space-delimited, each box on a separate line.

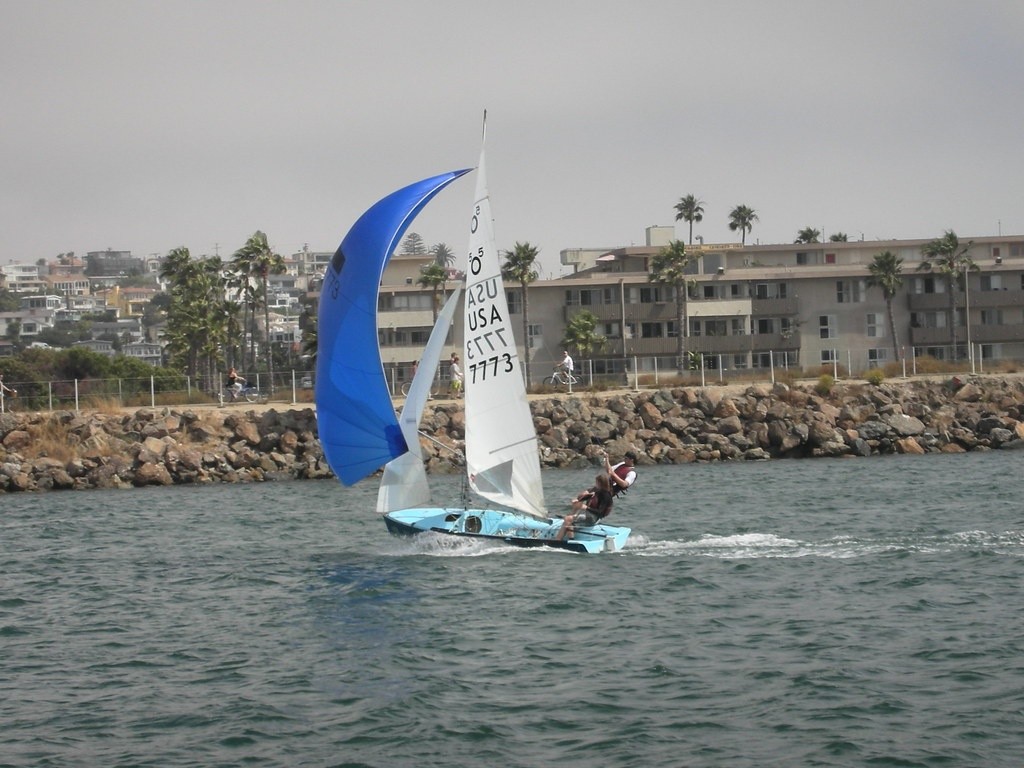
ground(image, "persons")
xmin=555 ymin=474 xmax=613 ymax=541
xmin=0 ymin=373 xmax=13 ymax=408
xmin=556 ymin=450 xmax=637 ymax=518
xmin=226 ymin=367 xmax=244 ymax=398
xmin=411 ymin=353 xmax=465 ymax=400
xmin=557 ymin=351 xmax=574 ymax=382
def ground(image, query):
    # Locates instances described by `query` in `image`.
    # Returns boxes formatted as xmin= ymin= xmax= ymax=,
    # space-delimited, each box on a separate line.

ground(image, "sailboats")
xmin=311 ymin=107 xmax=632 ymax=554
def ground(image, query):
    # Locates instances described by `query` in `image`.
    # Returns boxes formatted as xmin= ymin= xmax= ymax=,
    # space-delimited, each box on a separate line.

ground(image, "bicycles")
xmin=0 ymin=388 xmax=26 ymax=413
xmin=216 ymin=377 xmax=262 ymax=403
xmin=541 ymin=365 xmax=584 ymax=392
xmin=399 ymin=380 xmax=440 ymax=398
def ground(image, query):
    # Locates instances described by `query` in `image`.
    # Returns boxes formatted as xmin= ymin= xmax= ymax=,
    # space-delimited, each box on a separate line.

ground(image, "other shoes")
xmin=457 ymin=396 xmax=463 ymax=398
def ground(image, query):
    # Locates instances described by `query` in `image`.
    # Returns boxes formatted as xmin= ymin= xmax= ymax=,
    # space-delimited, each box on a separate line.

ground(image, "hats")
xmin=452 ymin=353 xmax=458 ymax=357
